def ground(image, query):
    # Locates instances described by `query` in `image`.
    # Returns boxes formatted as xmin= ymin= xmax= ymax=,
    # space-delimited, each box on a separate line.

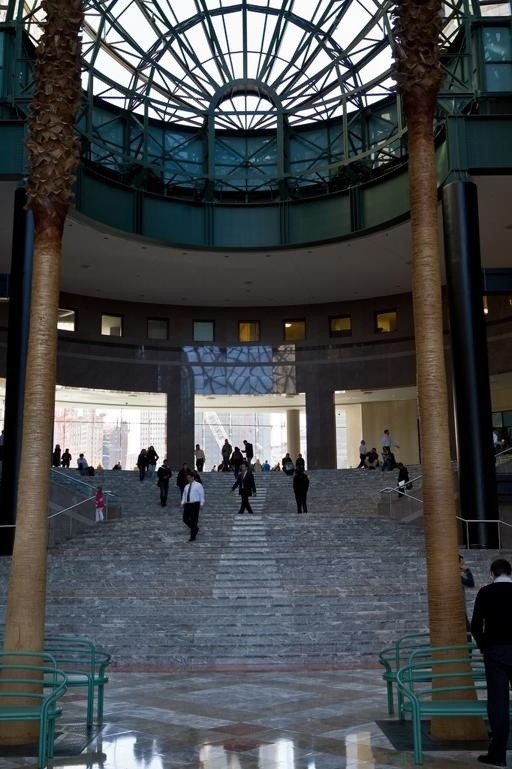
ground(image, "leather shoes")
xmin=477 ymin=754 xmax=506 ymax=766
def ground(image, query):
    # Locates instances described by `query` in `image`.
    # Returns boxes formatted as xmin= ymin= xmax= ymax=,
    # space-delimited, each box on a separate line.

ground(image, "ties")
xmin=187 ymin=484 xmax=191 ymax=502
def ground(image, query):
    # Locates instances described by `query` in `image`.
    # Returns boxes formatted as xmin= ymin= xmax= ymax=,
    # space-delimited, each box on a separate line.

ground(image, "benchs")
xmin=393 ymin=642 xmax=512 ymax=765
xmin=0 ymin=647 xmax=70 ymax=769
xmin=377 ymin=626 xmax=487 ymax=716
xmin=42 ymin=635 xmax=112 ymax=732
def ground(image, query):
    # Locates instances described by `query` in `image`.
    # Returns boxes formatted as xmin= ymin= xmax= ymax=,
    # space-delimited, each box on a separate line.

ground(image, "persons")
xmin=282 ymin=453 xmax=304 ymax=476
xmin=396 ymin=463 xmax=408 ymax=498
xmin=157 ymin=459 xmax=172 ymax=506
xmin=113 ymin=462 xmax=121 ymax=471
xmin=492 ymin=428 xmax=504 ymax=452
xmin=50 ymin=444 xmax=89 ymax=475
xmin=176 ymin=462 xmax=202 ymax=500
xmin=137 ymin=446 xmax=159 ymax=481
xmin=232 ymin=463 xmax=257 ymax=512
xmin=469 ymin=558 xmax=511 ymax=768
xmin=457 ymin=554 xmax=476 ymax=661
xmin=95 ymin=485 xmax=107 ymax=524
xmin=253 ymin=459 xmax=280 ymax=472
xmin=218 ymin=439 xmax=253 ymax=479
xmin=193 ymin=444 xmax=205 ymax=471
xmin=357 ymin=429 xmax=399 ymax=472
xmin=179 ymin=471 xmax=205 ymax=543
xmin=293 ymin=466 xmax=310 ymax=513
